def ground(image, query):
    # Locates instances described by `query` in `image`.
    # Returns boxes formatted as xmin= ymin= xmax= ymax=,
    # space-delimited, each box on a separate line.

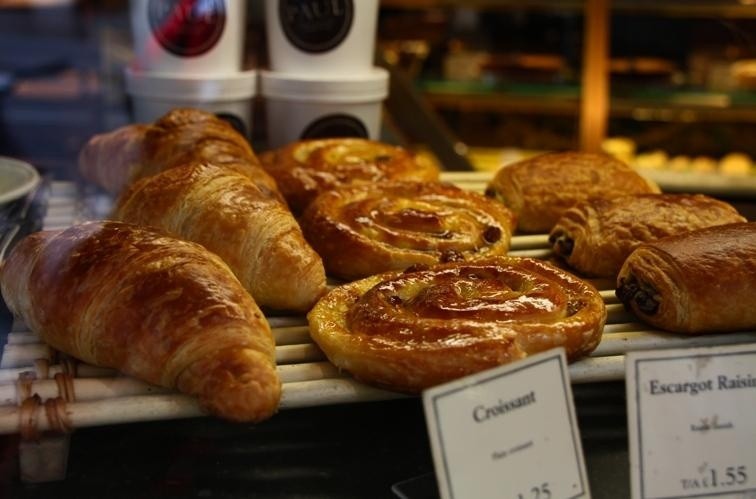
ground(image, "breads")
xmin=79 ymin=123 xmax=150 ymax=194
xmin=485 ymin=148 xmax=654 ymax=234
xmin=255 ymin=135 xmax=442 ymax=205
xmin=548 ymin=193 xmax=748 ymax=279
xmin=306 ymin=256 xmax=607 ymax=394
xmin=301 ymin=180 xmax=513 ymax=281
xmin=0 ymin=221 xmax=282 ymax=423
xmin=110 ymin=163 xmax=328 ymax=315
xmin=615 ymin=221 xmax=756 ymax=336
xmin=142 ymin=106 xmax=292 ymax=214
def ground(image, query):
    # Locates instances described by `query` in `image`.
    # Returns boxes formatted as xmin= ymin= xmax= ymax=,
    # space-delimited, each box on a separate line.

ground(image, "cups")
xmin=128 ymin=0 xmax=247 ymax=71
xmin=0 ymin=155 xmax=41 ymax=261
xmin=264 ymin=0 xmax=382 ymax=68
xmin=260 ymin=65 xmax=388 ymax=151
xmin=121 ymin=67 xmax=260 ymax=142
